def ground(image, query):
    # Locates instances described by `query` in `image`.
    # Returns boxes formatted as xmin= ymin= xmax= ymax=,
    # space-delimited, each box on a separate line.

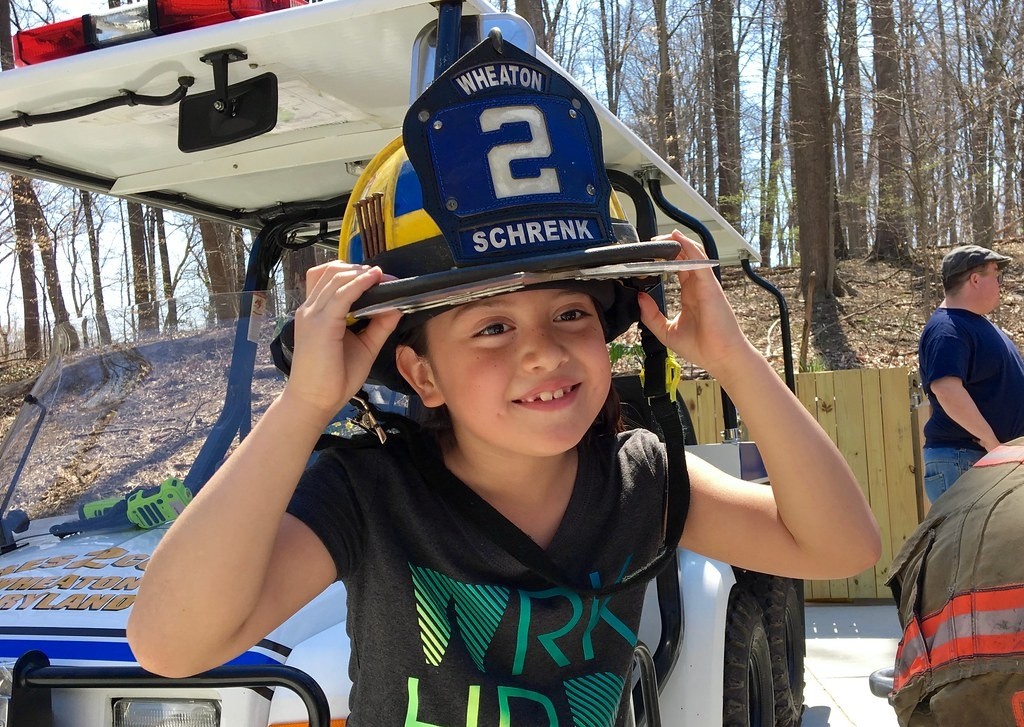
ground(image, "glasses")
xmin=978 ymin=271 xmax=1005 ymax=284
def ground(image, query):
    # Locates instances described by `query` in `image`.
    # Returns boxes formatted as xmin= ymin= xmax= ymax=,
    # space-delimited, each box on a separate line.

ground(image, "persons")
xmin=126 ymin=27 xmax=884 ymax=727
xmin=917 ymin=245 xmax=1024 ymax=504
xmin=884 ymin=434 xmax=1024 ymax=727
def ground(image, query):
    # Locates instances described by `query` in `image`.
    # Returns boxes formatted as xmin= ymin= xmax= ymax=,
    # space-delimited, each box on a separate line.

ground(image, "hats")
xmin=942 ymin=244 xmax=1012 ymax=279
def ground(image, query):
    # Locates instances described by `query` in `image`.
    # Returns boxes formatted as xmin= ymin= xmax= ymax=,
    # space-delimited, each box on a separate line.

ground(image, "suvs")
xmin=1 ymin=0 xmax=807 ymax=727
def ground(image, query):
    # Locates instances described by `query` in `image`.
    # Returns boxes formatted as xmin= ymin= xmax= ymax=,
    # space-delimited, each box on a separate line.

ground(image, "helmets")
xmin=278 ymin=26 xmax=738 ymax=385
